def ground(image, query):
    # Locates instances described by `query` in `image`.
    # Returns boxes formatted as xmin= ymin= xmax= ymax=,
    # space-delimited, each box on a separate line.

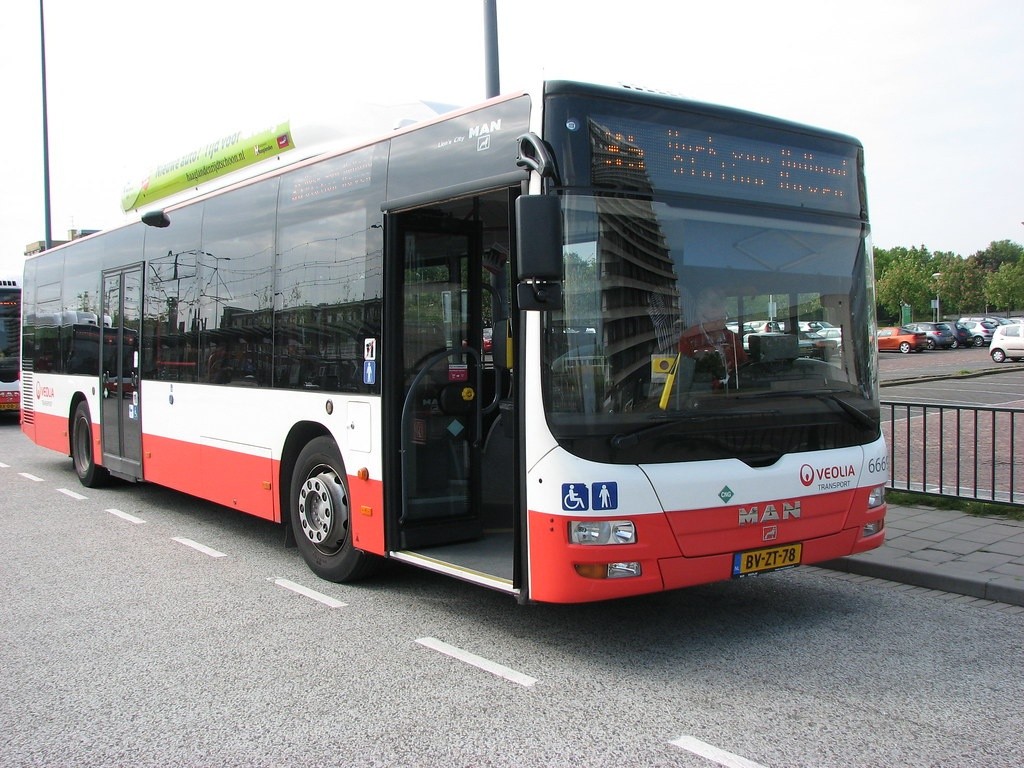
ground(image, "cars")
xmin=724 ymin=320 xmax=843 ymax=360
xmin=988 ymin=325 xmax=1023 ymax=363
xmin=482 ymin=327 xmax=493 ymax=353
xmin=877 ymin=327 xmax=928 ymax=354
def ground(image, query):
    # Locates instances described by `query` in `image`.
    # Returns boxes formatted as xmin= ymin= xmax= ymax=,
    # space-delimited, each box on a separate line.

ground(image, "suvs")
xmin=903 ymin=316 xmax=1024 ymax=351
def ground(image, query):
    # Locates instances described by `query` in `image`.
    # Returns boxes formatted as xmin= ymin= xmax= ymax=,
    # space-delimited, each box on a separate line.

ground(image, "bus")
xmin=0 ymin=279 xmax=22 ymax=418
xmin=22 ymin=310 xmax=137 ymax=397
xmin=20 ymin=80 xmax=891 ymax=615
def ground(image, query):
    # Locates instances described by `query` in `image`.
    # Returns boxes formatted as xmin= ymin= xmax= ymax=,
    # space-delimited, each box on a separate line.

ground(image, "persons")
xmin=678 ymin=286 xmax=748 ymax=390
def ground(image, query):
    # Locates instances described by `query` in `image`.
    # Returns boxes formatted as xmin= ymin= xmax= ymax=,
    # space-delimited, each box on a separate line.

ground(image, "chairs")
xmin=648 ymin=283 xmax=696 ymax=354
xmin=207 ymin=350 xmax=235 ymax=384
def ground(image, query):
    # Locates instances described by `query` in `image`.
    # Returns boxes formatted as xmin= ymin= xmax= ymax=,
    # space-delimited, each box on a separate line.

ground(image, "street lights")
xmin=932 ymin=273 xmax=943 ymax=322
xmin=206 ymin=252 xmax=231 ymax=329
